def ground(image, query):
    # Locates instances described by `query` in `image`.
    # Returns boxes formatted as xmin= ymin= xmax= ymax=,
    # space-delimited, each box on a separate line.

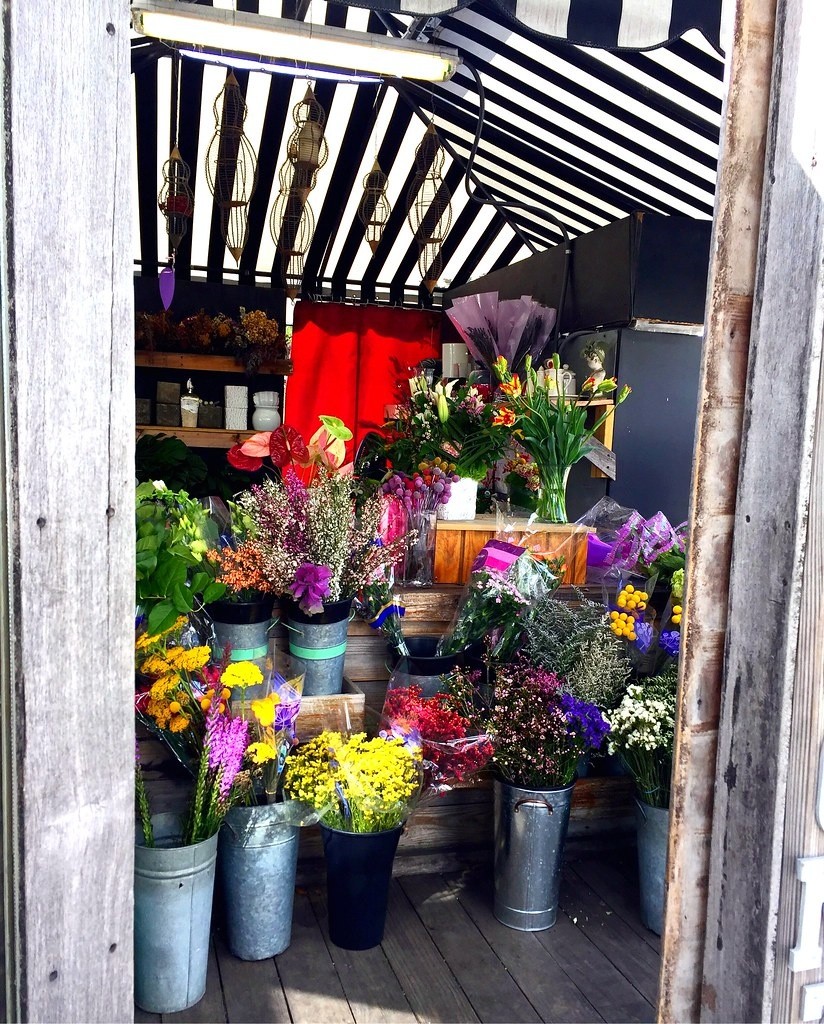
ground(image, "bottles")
xmin=563 ymin=364 xmax=576 ymax=395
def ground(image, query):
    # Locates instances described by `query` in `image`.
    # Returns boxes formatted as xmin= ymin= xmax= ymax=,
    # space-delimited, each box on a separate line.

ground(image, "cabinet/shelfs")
xmin=134 ymin=271 xmax=294 ymax=450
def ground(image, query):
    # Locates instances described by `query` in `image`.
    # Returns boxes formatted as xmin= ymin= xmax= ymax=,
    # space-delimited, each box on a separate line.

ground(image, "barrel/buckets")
xmin=383 ymin=634 xmax=472 ymax=698
xmin=632 ymin=791 xmax=670 ymax=938
xmin=220 ymin=799 xmax=300 ymax=961
xmin=319 ymin=820 xmax=407 ymax=950
xmin=134 ymin=812 xmax=220 ymax=1013
xmin=282 ymin=598 xmax=355 ymax=696
xmin=212 ymin=597 xmax=281 ymax=700
xmin=491 ymin=770 xmax=578 ymax=931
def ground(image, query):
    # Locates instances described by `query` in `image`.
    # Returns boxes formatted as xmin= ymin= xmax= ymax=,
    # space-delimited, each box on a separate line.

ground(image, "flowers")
xmin=132 ymin=290 xmax=689 ymax=852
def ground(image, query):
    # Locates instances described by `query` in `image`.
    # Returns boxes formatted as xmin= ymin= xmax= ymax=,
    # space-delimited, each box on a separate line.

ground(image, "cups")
xmin=545 ymin=369 xmax=571 ymax=396
xmin=414 ymin=368 xmax=435 ymax=390
xmin=528 ymin=371 xmax=544 ymax=395
